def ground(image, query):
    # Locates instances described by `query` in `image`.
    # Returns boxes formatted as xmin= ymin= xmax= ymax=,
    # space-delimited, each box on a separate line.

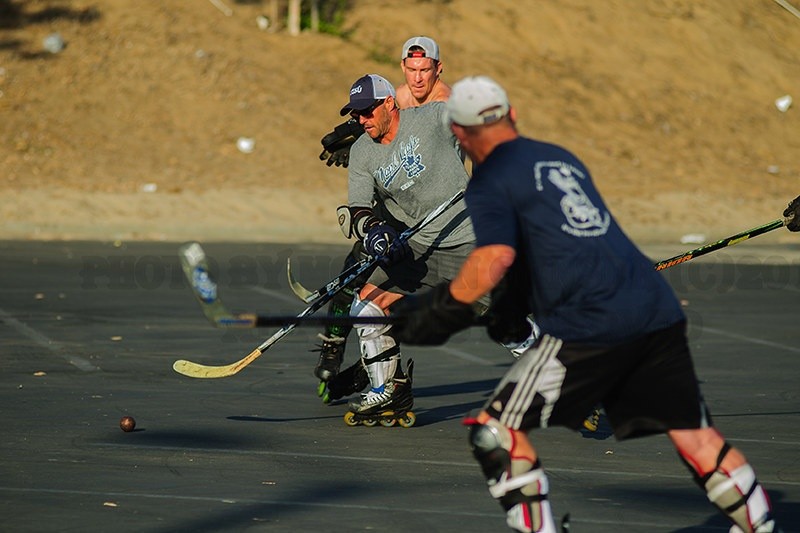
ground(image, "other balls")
xmin=120 ymin=416 xmax=136 ymax=432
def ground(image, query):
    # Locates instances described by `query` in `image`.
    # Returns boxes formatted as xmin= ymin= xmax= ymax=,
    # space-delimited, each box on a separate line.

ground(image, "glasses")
xmin=350 ymin=99 xmax=386 ymax=121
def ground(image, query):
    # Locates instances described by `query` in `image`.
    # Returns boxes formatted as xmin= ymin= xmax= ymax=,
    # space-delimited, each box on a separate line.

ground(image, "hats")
xmin=448 ymin=75 xmax=509 ymax=127
xmin=347 ymin=75 xmax=395 ymax=110
xmin=401 ymin=37 xmax=443 ymax=73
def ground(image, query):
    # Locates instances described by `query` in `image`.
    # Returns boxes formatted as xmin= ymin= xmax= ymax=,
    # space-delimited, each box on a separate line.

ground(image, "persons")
xmin=336 ymin=74 xmax=542 ymax=427
xmin=313 ymin=35 xmax=453 ymax=402
xmin=389 ymin=74 xmax=785 ymax=533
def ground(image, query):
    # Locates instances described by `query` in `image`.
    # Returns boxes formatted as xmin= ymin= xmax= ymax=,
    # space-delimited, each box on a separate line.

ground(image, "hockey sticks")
xmin=286 ymin=257 xmax=375 ymax=304
xmin=653 ymin=213 xmax=794 ymax=272
xmin=177 ymin=241 xmax=489 ymax=327
xmin=172 ymin=189 xmax=468 ymax=379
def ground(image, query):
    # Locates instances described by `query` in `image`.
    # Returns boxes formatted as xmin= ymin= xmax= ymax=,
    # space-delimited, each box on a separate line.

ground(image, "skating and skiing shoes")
xmin=344 ymin=358 xmax=416 ymax=430
xmin=318 ymin=358 xmax=371 ymax=404
xmin=313 ymin=338 xmax=347 ymax=397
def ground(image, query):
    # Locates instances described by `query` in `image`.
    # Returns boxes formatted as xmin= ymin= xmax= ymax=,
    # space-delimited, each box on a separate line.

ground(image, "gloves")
xmin=363 ymin=222 xmax=409 ymax=267
xmin=383 ymin=279 xmax=476 ymax=347
xmin=783 ymin=195 xmax=800 ymax=233
